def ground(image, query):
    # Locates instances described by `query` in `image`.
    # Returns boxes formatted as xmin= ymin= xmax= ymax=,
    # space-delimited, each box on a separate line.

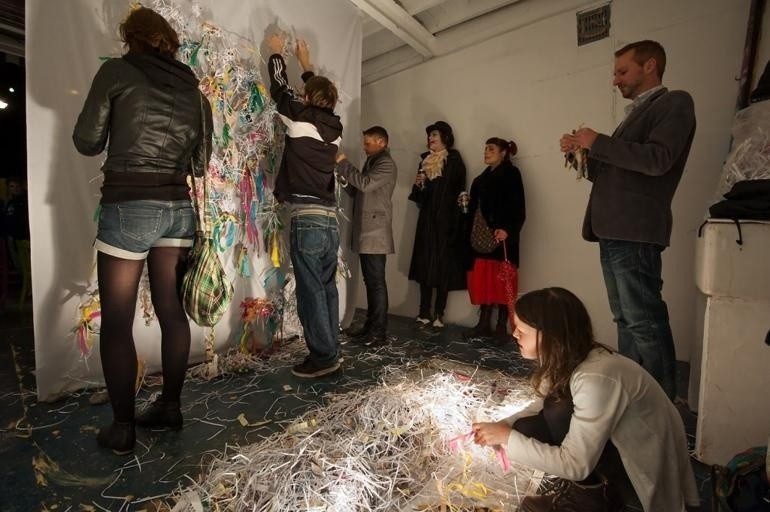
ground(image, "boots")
xmin=522 ymin=471 xmax=611 ymax=512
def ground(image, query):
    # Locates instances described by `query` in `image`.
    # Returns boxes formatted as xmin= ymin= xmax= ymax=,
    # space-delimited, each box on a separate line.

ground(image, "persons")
xmin=406 ymin=120 xmax=467 ymax=332
xmin=471 ymin=286 xmax=702 ymax=512
xmin=560 ymin=38 xmax=696 ymax=401
xmin=459 ymin=137 xmax=526 ymax=345
xmin=71 ymin=7 xmax=214 ymax=457
xmin=3 ymin=178 xmax=33 ymax=298
xmin=337 ymin=126 xmax=397 ymax=348
xmin=267 ymin=33 xmax=344 ymax=377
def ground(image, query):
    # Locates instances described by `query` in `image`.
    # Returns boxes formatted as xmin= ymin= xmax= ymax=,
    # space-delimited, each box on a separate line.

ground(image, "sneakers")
xmin=411 ymin=317 xmax=443 ymax=336
xmin=351 ymin=329 xmax=387 ymax=347
xmin=107 ymin=419 xmax=136 ymax=456
xmin=134 ymin=395 xmax=185 ymax=431
xmin=462 ymin=327 xmax=506 ymax=348
xmin=290 ymin=352 xmax=346 ymax=379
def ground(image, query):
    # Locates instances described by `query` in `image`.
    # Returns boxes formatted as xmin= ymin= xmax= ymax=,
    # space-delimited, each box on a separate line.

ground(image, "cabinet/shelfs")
xmin=687 ymin=218 xmax=770 ymax=470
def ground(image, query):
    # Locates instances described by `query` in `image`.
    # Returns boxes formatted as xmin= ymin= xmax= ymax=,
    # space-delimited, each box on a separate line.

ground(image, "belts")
xmin=289 ymin=209 xmax=338 ymax=219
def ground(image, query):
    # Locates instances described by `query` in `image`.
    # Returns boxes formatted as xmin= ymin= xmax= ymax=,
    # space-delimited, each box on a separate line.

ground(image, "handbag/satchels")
xmin=710 ymin=446 xmax=770 ymax=512
xmin=180 ymin=225 xmax=236 ymax=329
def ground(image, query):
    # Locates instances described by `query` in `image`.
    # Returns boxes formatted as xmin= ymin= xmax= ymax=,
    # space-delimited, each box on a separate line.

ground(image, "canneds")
xmin=460 ymin=192 xmax=469 ymax=214
xmin=418 ymin=169 xmax=426 ymax=191
